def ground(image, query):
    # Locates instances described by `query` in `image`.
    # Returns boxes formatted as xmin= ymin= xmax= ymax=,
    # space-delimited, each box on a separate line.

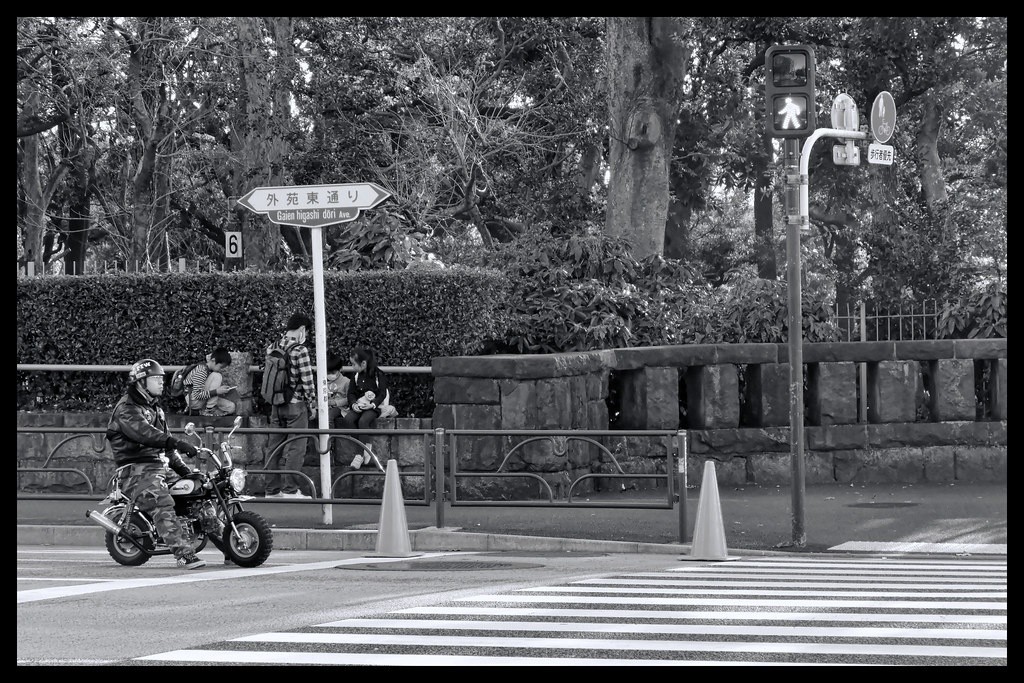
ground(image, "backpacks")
xmin=355 ymin=370 xmax=390 ymax=415
xmin=170 ymin=363 xmax=206 ymax=414
xmin=261 ymin=339 xmax=299 ymax=404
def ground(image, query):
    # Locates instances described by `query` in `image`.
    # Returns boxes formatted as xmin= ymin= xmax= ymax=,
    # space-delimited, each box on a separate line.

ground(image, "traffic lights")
xmin=765 ymin=45 xmax=815 ymax=135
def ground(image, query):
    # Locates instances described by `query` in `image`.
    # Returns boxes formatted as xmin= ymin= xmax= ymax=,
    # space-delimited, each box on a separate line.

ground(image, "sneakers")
xmin=177 ymin=554 xmax=206 ymax=570
xmin=265 ymin=490 xmax=312 ymax=499
xmin=364 ymin=444 xmax=372 ymax=464
xmin=350 ymin=453 xmax=363 ymax=469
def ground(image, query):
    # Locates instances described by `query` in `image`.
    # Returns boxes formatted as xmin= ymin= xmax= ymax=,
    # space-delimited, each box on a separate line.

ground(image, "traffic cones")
xmin=358 ymin=459 xmax=420 ymax=559
xmin=674 ymin=458 xmax=744 ymax=564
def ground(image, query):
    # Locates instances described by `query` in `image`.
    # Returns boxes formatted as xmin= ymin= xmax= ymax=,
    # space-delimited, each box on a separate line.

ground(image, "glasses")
xmin=148 ymin=375 xmax=162 ymax=381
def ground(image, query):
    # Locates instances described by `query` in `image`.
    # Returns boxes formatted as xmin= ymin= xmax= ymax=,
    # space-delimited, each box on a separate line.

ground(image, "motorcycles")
xmin=86 ymin=414 xmax=274 ymax=567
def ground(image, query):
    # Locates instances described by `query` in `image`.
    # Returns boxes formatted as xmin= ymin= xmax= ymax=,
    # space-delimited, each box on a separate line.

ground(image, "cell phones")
xmin=328 ymin=392 xmax=333 ymax=397
xmin=227 ymin=386 xmax=237 ymax=391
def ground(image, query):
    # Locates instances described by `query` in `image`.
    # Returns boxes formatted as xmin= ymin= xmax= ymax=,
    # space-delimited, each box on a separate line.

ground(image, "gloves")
xmin=178 ymin=439 xmax=197 ymax=459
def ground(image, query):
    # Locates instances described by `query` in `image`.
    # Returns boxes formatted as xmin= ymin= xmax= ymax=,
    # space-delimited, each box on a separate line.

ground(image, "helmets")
xmin=128 ymin=359 xmax=164 ymax=382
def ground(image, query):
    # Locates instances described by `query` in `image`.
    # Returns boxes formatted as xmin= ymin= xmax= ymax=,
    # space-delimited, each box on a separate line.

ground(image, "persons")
xmin=183 ymin=348 xmax=236 ymax=415
xmin=106 ymin=359 xmax=236 ymax=569
xmin=345 ymin=347 xmax=389 ymax=471
xmin=264 ymin=313 xmax=316 ymax=499
xmin=306 ymin=354 xmax=349 ymax=457
